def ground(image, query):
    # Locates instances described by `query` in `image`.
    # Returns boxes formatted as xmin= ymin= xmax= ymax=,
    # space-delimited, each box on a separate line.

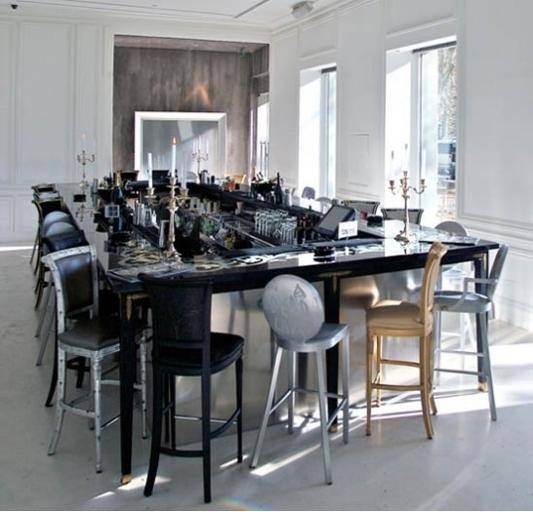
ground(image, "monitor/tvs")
xmin=313 ymin=204 xmax=356 ymax=238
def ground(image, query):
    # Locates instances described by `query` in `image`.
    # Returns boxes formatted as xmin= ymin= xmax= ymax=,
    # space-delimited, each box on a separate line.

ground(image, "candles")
xmin=167 ymin=137 xmax=179 ymax=173
xmin=388 ymin=141 xmax=426 ymax=181
xmin=144 ymin=151 xmax=157 ymax=188
xmin=189 ymin=132 xmax=210 ymax=154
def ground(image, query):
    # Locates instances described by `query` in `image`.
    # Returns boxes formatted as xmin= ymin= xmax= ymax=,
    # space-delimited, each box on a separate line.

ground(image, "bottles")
xmin=297 ymin=205 xmax=319 ymax=245
xmin=132 ymin=199 xmax=145 ymax=226
xmin=91 ymin=169 xmax=122 ymax=195
xmin=199 ymin=168 xmax=235 ymax=192
xmin=266 ymin=172 xmax=293 ymax=206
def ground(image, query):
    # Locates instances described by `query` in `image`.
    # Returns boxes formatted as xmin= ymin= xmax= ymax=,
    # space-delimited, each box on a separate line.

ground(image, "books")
xmin=105 ymin=261 xmax=192 ymax=284
xmin=418 ymin=235 xmax=481 ymax=246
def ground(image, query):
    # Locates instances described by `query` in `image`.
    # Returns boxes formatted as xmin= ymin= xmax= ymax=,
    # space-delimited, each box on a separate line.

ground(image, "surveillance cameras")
xmin=11 ymin=4 xmax=18 ymax=9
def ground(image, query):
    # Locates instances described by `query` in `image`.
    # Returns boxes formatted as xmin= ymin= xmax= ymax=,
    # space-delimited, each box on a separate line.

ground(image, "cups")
xmin=234 ymin=202 xmax=243 ymax=215
xmin=189 ymin=195 xmax=220 ymax=213
xmin=252 ymin=207 xmax=295 ymax=242
